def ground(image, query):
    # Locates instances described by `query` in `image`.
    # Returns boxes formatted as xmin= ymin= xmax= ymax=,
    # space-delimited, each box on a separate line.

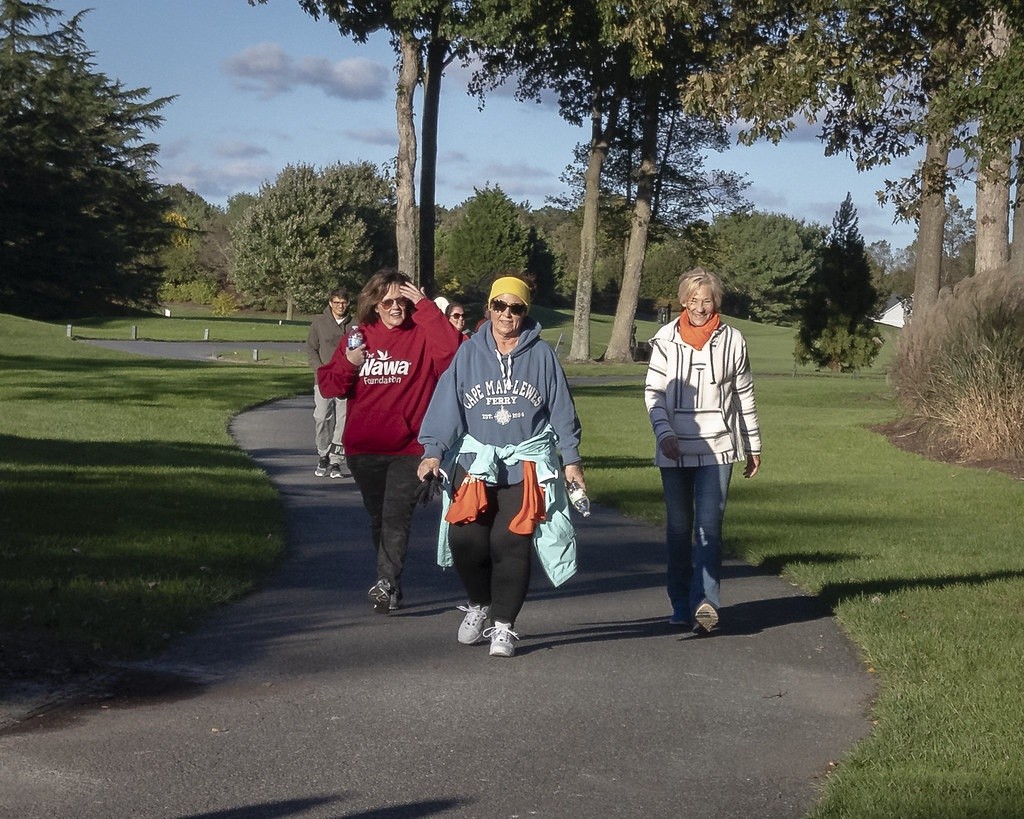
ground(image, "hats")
xmin=486 ymin=277 xmax=534 ymax=316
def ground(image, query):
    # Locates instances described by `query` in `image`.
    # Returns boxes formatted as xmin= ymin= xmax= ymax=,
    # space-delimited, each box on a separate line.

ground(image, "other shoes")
xmin=691 ymin=599 xmax=719 ymax=635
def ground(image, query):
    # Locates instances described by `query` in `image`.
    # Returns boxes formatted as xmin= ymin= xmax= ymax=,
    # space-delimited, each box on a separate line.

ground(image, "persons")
xmin=317 ymin=267 xmax=470 ymax=614
xmin=416 ymin=275 xmax=587 ymax=658
xmin=644 ymin=266 xmax=762 ymax=637
xmin=305 ymin=287 xmax=359 ymax=478
xmin=434 ymin=293 xmax=494 ymax=347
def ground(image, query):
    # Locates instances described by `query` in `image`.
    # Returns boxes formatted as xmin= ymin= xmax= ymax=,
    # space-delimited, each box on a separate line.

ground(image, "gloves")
xmin=414 ymin=470 xmax=445 ymax=509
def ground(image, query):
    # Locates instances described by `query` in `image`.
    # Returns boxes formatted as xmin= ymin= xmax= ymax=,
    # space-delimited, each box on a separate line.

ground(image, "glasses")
xmin=490 ymin=297 xmax=528 ymax=316
xmin=376 ymin=296 xmax=407 ymax=310
xmin=330 ymin=300 xmax=348 ymax=305
xmin=450 ymin=314 xmax=466 ymax=319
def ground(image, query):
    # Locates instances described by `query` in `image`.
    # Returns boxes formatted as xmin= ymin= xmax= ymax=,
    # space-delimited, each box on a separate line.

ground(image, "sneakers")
xmin=328 ymin=466 xmax=344 ymax=479
xmin=314 ymin=457 xmax=328 ymax=477
xmin=457 ymin=601 xmax=493 ymax=644
xmin=387 ymin=589 xmax=400 ymax=610
xmin=488 ymin=621 xmax=516 ymax=657
xmin=366 ymin=578 xmax=393 ymax=615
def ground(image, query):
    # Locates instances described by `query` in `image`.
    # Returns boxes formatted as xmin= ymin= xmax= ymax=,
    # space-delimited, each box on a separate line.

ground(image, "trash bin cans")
xmin=637 ymin=341 xmax=651 ymax=361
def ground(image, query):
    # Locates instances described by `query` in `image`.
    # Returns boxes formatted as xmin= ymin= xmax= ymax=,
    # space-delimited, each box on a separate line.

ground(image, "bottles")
xmin=347 ymin=325 xmax=364 ymax=352
xmin=564 ymin=480 xmax=590 ymax=518
